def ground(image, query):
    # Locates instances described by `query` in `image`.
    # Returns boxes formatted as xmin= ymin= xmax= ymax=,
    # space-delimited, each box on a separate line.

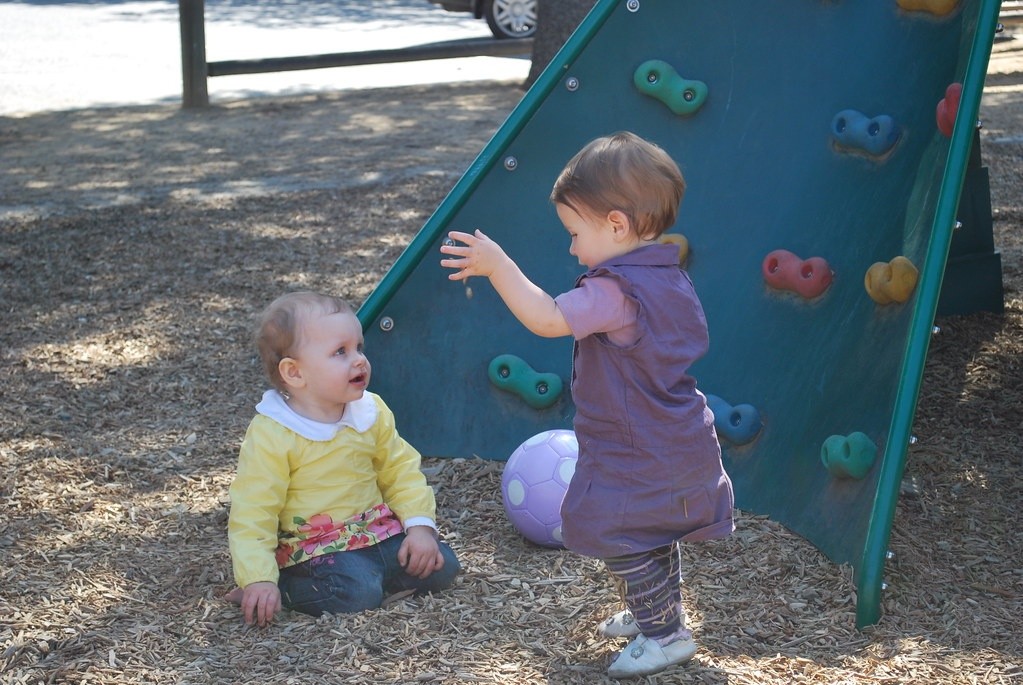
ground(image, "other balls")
xmin=501 ymin=429 xmax=579 ymax=550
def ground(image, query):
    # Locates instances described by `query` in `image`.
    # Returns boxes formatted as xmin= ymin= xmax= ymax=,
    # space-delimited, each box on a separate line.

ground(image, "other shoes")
xmin=598 ymin=608 xmax=640 ymax=636
xmin=608 ymin=634 xmax=697 ymax=677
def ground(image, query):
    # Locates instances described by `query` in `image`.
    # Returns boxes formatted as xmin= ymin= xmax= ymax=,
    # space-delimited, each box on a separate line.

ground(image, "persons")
xmin=228 ymin=291 xmax=460 ymax=628
xmin=439 ymin=129 xmax=735 ymax=677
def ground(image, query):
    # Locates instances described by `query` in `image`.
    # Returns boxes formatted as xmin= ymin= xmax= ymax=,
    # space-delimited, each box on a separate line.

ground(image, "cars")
xmin=428 ymin=0 xmax=538 ymax=39
